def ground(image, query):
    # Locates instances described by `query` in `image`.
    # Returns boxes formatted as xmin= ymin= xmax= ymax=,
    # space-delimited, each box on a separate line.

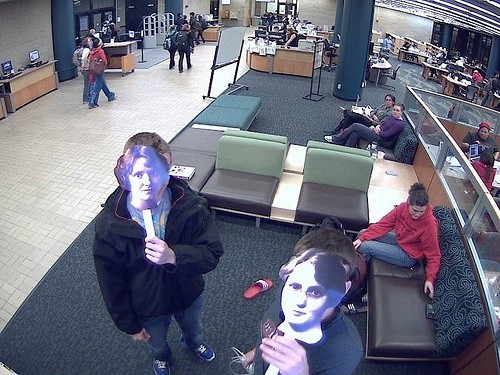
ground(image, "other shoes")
xmin=89 ymin=104 xmax=98 ymax=109
xmin=324 ymin=136 xmax=333 ymax=142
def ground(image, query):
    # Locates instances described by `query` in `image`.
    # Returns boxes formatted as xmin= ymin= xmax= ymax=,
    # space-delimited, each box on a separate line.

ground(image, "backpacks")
xmin=164 ymin=32 xmax=178 ymax=50
xmin=176 ymin=31 xmax=190 ymax=49
xmin=72 ymin=47 xmax=90 ymax=67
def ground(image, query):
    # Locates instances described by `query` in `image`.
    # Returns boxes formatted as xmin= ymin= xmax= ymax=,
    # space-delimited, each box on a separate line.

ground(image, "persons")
xmin=276 ymin=248 xmax=347 ymax=344
xmin=466 ymin=150 xmax=496 ymax=195
xmin=167 ymin=24 xmax=194 ymax=72
xmin=114 ymin=146 xmax=168 ymax=211
xmin=189 ymin=12 xmax=200 ymax=46
xmin=92 ymin=131 xmax=224 ymax=375
xmin=383 ymin=36 xmax=392 ymax=59
xmin=462 ymin=122 xmax=500 ymax=160
xmin=428 ymin=51 xmax=483 ymax=81
xmin=286 ymin=28 xmax=297 ymax=47
xmin=242 ymin=227 xmax=364 ymax=375
xmin=267 ymin=12 xmax=274 ymax=31
xmin=79 ymin=36 xmax=116 ymax=109
xmin=100 ymin=20 xmax=117 ymax=42
xmin=86 ymin=29 xmax=95 ymax=38
xmin=198 ymin=16 xmax=206 ymax=45
xmin=332 ymin=94 xmax=394 ymax=135
xmin=325 ymin=104 xmax=405 ymax=151
xmin=353 ymin=183 xmax=441 ymax=298
xmin=482 ymin=71 xmax=499 ymax=108
xmin=177 ymin=14 xmax=188 ymax=27
xmin=403 ymin=42 xmax=418 ymax=62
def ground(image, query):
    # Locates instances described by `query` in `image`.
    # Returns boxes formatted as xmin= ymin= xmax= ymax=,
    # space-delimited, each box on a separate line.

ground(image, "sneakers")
xmin=153 ymin=357 xmax=170 ymax=375
xmin=193 ymin=342 xmax=215 ymax=361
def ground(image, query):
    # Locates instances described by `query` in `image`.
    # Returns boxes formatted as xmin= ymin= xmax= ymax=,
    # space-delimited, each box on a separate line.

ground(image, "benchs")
xmin=154 ymin=95 xmax=488 ymax=364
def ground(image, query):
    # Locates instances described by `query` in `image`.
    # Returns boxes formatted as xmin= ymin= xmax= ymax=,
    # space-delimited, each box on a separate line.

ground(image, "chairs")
xmin=323 ymin=38 xmax=338 ymax=72
xmin=337 ymin=33 xmax=343 ymax=42
xmin=378 ymin=63 xmax=402 ymax=92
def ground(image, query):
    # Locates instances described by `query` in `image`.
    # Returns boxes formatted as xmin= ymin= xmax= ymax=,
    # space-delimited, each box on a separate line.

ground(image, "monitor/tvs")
xmin=1 ymin=60 xmax=12 ymax=72
xmin=29 ymin=49 xmax=40 ymax=61
xmin=469 ymin=143 xmax=478 ymax=157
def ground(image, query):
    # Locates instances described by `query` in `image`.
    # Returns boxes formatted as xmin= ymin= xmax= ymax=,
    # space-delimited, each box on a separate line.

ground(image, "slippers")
xmin=341 ymin=301 xmax=367 ymax=314
xmin=243 ymin=279 xmax=272 ymax=299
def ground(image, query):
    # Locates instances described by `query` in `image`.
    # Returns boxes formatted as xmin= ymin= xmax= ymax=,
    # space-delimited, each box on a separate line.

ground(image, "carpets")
xmin=0 ymin=47 xmax=500 ymax=375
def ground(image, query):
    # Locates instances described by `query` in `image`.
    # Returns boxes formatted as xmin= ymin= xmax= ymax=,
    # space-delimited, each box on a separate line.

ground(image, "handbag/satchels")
xmin=365 ymin=105 xmax=373 ymax=118
xmin=89 ymin=50 xmax=104 ymax=76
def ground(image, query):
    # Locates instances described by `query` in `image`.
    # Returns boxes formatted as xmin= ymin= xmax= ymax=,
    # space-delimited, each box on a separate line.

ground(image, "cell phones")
xmin=426 ymin=302 xmax=434 ymax=319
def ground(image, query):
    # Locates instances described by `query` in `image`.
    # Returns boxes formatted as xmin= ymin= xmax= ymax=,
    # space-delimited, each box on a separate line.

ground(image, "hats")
xmin=479 ymin=122 xmax=491 ymax=130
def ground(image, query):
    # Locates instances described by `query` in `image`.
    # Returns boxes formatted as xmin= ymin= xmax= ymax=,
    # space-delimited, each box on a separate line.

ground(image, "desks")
xmin=323 ymin=28 xmax=500 ymax=107
xmin=0 ymin=38 xmax=142 ymax=122
